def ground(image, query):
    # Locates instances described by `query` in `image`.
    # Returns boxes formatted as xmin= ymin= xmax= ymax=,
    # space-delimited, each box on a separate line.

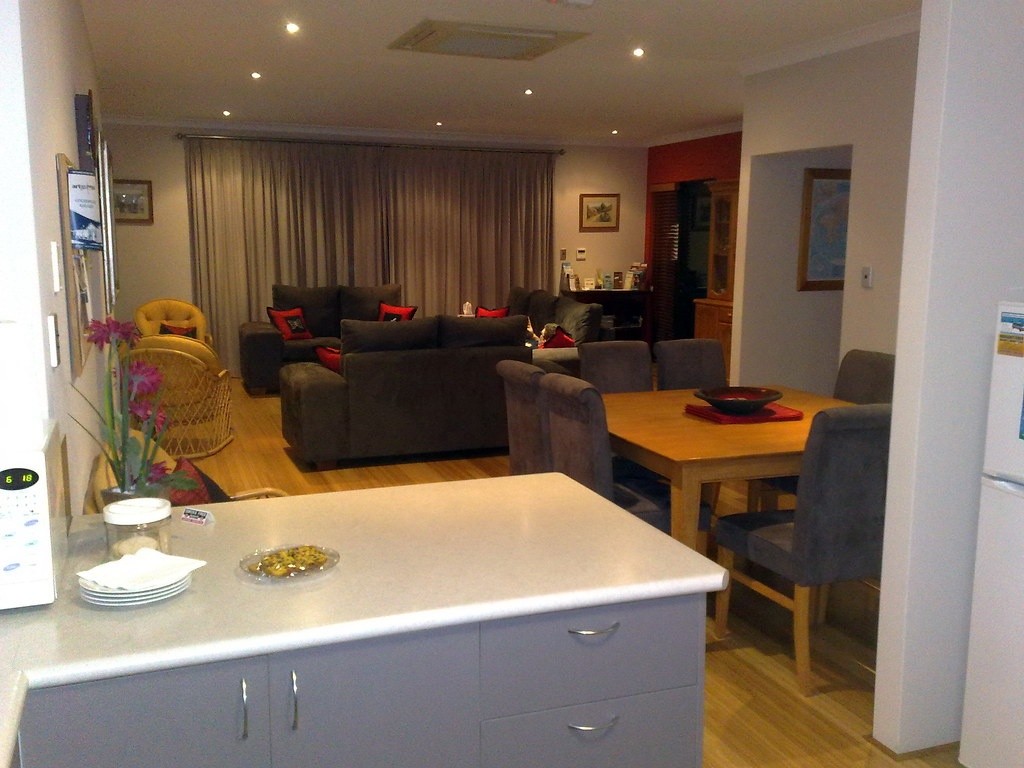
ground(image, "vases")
xmin=101 ymin=483 xmax=172 ymax=506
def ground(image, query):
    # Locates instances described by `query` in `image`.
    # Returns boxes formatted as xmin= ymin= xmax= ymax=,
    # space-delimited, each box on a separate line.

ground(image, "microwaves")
xmin=0 ymin=418 xmax=68 ymax=610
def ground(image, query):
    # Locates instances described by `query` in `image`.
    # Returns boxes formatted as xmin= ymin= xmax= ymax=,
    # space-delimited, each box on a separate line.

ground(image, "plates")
xmin=239 ymin=544 xmax=340 ymax=584
xmin=78 ymin=572 xmax=192 ymax=607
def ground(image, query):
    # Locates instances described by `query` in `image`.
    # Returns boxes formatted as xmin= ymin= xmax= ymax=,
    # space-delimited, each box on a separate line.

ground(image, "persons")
xmin=527 ymin=316 xmax=565 ymax=349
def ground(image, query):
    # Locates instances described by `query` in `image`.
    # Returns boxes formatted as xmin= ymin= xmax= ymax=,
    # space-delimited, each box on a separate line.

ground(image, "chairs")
xmin=87 ymin=426 xmax=288 ymax=513
xmin=131 ymin=299 xmax=234 ymax=459
xmin=494 ymin=338 xmax=895 ymax=697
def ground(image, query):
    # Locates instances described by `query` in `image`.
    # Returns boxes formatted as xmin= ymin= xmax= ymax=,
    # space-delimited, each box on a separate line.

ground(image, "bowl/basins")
xmin=693 ymin=386 xmax=784 ymax=416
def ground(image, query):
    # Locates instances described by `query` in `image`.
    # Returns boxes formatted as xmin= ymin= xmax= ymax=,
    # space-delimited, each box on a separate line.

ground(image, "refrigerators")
xmin=957 ymin=300 xmax=1024 ymax=768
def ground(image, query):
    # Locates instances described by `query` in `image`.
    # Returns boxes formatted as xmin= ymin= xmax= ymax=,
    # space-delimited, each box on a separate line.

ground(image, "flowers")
xmin=64 ymin=317 xmax=197 ymax=497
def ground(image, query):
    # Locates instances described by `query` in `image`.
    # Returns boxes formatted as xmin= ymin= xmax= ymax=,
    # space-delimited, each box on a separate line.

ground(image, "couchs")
xmin=279 ymin=315 xmax=532 ymax=471
xmin=238 ymin=283 xmax=401 ymax=397
xmin=508 ymin=286 xmax=602 ymax=374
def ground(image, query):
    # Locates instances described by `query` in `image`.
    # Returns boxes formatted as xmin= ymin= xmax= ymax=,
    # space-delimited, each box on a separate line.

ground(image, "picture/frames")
xmin=692 ymin=192 xmax=711 ymax=231
xmin=112 ymin=179 xmax=154 ymax=225
xmin=579 ymin=194 xmax=620 ymax=233
xmin=797 ymin=167 xmax=851 ymax=291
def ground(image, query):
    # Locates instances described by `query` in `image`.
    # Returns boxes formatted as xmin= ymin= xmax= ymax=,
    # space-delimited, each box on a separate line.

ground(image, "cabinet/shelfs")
xmin=692 ymin=178 xmax=739 ymax=384
xmin=19 ymin=592 xmax=707 ymax=768
xmin=561 ymin=289 xmax=643 ymax=340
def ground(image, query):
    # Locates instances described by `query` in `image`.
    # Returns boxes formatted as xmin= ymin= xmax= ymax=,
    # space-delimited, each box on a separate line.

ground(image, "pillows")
xmin=312 ymin=344 xmax=340 ymax=373
xmin=539 ymin=322 xmax=576 ymax=348
xmin=159 ymin=322 xmax=197 ymax=339
xmin=157 ymin=458 xmax=230 ymax=506
xmin=378 ymin=300 xmax=418 ymax=320
xmin=266 ymin=305 xmax=312 ymax=340
xmin=475 ymin=307 xmax=510 ymax=318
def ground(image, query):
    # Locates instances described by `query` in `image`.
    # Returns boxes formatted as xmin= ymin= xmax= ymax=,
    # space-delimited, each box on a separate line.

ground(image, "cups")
xmin=102 ymin=497 xmax=173 ymax=561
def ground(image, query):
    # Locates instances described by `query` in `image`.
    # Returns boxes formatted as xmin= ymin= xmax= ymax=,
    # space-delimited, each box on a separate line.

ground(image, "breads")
xmin=246 ymin=544 xmax=328 ymax=577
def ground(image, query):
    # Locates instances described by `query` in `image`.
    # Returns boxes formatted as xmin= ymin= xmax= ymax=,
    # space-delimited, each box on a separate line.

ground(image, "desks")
xmin=599 ymin=385 xmax=858 ymax=552
